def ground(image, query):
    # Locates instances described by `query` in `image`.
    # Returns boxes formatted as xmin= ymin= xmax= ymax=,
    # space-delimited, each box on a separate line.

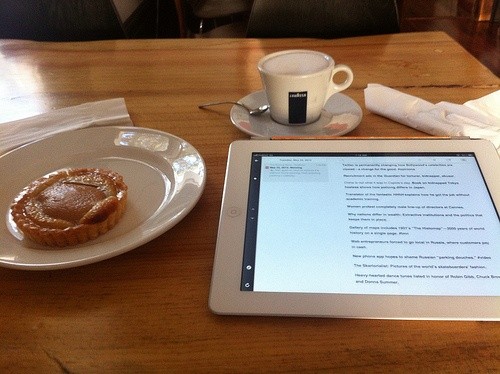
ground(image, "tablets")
xmin=209 ymin=139 xmax=499 ymax=323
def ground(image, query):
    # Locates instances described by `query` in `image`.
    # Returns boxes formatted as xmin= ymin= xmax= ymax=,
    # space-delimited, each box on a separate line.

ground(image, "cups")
xmin=256 ymin=49 xmax=355 ymax=127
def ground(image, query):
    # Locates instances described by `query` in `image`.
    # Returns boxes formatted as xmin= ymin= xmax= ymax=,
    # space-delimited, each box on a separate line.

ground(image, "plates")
xmin=0 ymin=124 xmax=206 ymax=272
xmin=230 ymin=87 xmax=362 ymax=138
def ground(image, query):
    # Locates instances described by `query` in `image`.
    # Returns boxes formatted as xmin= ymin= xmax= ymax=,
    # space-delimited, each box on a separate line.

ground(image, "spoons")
xmin=198 ymin=99 xmax=270 ymax=116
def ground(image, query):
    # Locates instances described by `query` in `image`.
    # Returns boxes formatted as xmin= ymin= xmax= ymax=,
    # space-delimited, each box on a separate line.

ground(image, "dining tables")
xmin=0 ymin=30 xmax=500 ymax=374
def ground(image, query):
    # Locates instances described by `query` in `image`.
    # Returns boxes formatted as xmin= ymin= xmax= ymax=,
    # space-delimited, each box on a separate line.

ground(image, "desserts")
xmin=9 ymin=166 xmax=128 ymax=249
